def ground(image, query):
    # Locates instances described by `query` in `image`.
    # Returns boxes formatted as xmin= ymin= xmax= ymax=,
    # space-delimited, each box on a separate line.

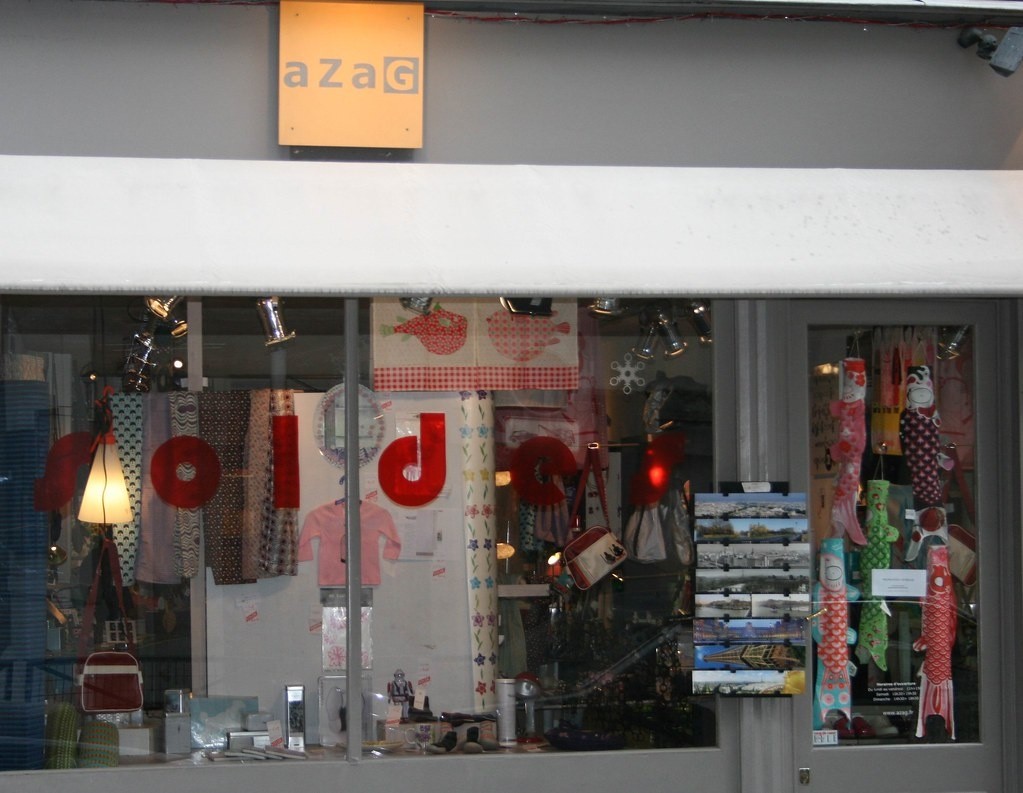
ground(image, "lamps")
xmin=144 ymin=296 xmax=187 ymax=337
xmin=398 ymin=296 xmax=432 ymax=314
xmin=498 ymin=296 xmax=553 ymax=317
xmin=79 ymin=386 xmax=132 ymax=523
xmin=589 ymin=298 xmax=714 ymax=358
xmin=257 ymin=295 xmax=295 ymax=347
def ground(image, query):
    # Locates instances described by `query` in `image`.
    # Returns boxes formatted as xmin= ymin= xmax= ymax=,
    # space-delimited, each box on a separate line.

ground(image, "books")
xmin=207 ymin=748 xmax=307 ymax=762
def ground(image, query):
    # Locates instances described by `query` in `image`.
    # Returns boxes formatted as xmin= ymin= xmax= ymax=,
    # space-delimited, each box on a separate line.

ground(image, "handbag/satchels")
xmin=623 ymin=502 xmax=695 ymax=565
xmin=564 ymin=445 xmax=628 ymax=591
xmin=78 ymin=539 xmax=144 ymax=713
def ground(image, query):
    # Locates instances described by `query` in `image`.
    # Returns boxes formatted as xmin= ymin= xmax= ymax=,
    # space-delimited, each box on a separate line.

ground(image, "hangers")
xmin=335 ymin=474 xmax=362 ymax=507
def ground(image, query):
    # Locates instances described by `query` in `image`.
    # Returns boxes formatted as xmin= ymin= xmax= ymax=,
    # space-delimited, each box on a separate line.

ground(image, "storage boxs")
xmin=226 ymin=731 xmax=269 ymax=751
xmin=242 ymin=713 xmax=274 ymax=732
xmin=117 ymin=727 xmax=162 ymax=754
xmin=191 ymin=695 xmax=259 ymax=747
xmin=379 ymin=721 xmax=498 ymax=750
xmin=317 ymin=675 xmax=372 ymax=746
xmin=148 ymin=716 xmax=192 ymax=754
